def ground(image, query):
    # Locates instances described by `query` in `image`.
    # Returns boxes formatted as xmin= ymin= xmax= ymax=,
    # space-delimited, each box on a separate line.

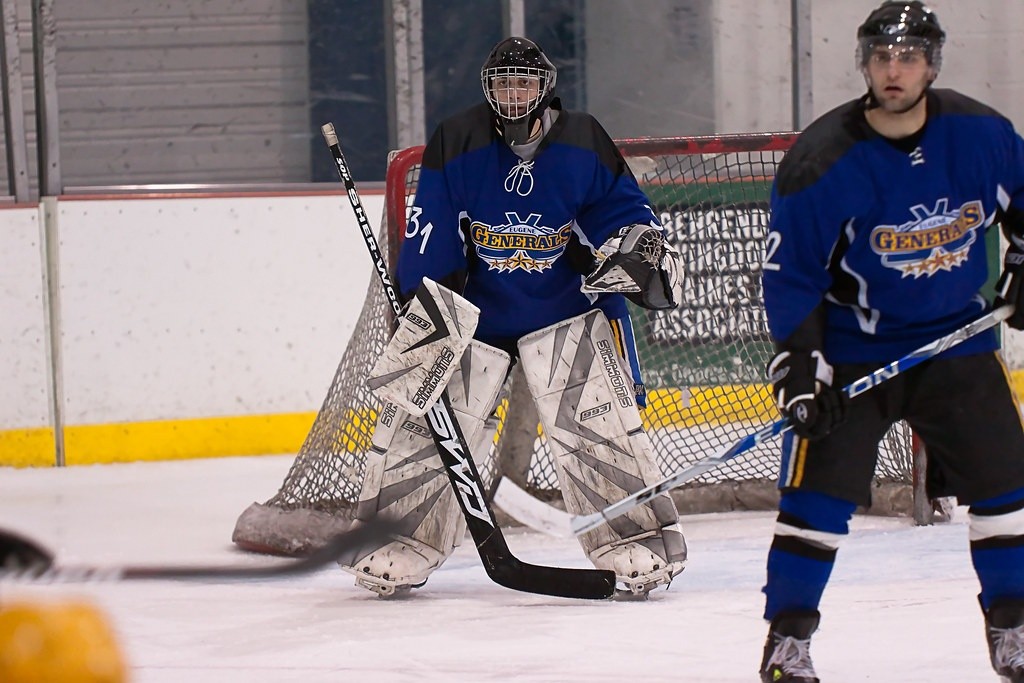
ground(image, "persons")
xmin=758 ymin=0 xmax=1024 ymax=683
xmin=331 ymin=37 xmax=688 ymax=593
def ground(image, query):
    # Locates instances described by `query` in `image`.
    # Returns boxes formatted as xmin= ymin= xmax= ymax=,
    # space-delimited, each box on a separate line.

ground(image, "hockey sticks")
xmin=321 ymin=123 xmax=620 ymax=600
xmin=486 ymin=290 xmax=1014 ymax=543
xmin=0 ymin=490 xmax=405 ymax=596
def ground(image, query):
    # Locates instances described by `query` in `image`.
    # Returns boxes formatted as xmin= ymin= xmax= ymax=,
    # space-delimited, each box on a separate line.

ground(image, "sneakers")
xmin=978 ymin=592 xmax=1024 ymax=683
xmin=760 ymin=612 xmax=820 ymax=683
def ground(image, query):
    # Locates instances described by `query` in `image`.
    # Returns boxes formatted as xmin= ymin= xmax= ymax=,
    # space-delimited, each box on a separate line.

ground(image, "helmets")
xmin=854 ymin=0 xmax=946 ymax=67
xmin=480 ymin=35 xmax=557 ymax=118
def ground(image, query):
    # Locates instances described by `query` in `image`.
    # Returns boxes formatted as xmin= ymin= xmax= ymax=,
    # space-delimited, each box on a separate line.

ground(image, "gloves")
xmin=993 ymin=234 xmax=1024 ymax=330
xmin=766 ymin=349 xmax=845 ymax=441
